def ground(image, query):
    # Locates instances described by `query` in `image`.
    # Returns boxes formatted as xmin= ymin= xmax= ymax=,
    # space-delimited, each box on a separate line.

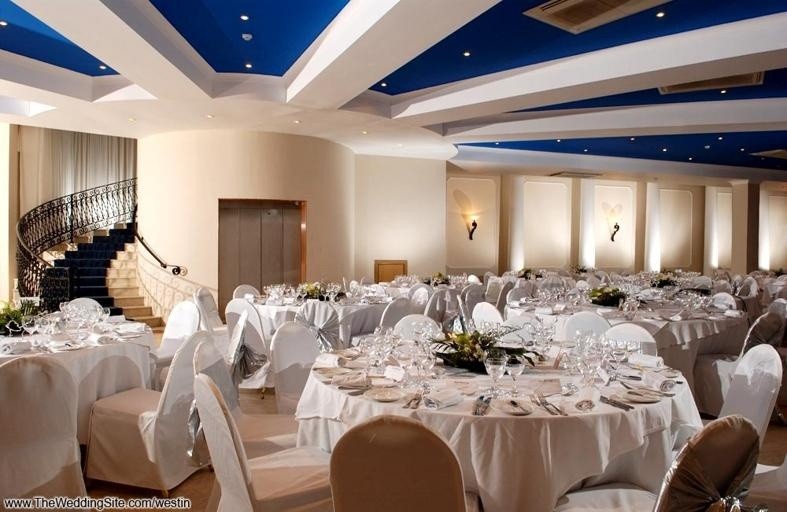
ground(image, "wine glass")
xmin=262 ymin=265 xmax=778 ymax=314
xmin=363 ymin=325 xmax=638 ymax=401
xmin=22 ymin=301 xmax=126 ymax=354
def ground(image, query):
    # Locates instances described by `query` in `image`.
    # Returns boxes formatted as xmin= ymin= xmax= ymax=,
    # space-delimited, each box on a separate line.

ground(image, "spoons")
xmin=508 ymin=401 xmax=528 ymax=413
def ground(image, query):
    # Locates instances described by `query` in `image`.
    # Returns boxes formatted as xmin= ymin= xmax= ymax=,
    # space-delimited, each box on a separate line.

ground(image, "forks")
xmin=532 ymin=390 xmax=568 ymax=420
xmin=401 ymin=388 xmax=425 ymax=410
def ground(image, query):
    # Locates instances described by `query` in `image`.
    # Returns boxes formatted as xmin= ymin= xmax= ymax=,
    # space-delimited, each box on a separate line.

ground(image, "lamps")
xmin=463 ymin=210 xmax=481 ymax=240
xmin=605 ymin=211 xmax=623 ymax=242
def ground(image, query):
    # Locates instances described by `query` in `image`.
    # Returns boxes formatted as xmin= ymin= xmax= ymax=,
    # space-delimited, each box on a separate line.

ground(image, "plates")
xmin=624 ymin=388 xmax=663 ymax=405
xmin=312 ymin=366 xmax=353 ymax=378
xmin=489 ymin=396 xmax=534 ymax=416
xmin=650 ymin=364 xmax=682 ymax=379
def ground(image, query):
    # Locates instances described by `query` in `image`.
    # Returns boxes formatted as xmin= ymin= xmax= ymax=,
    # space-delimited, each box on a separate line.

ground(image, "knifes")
xmin=598 ymin=397 xmax=636 ymax=414
xmin=472 ymin=396 xmax=493 ymax=417
